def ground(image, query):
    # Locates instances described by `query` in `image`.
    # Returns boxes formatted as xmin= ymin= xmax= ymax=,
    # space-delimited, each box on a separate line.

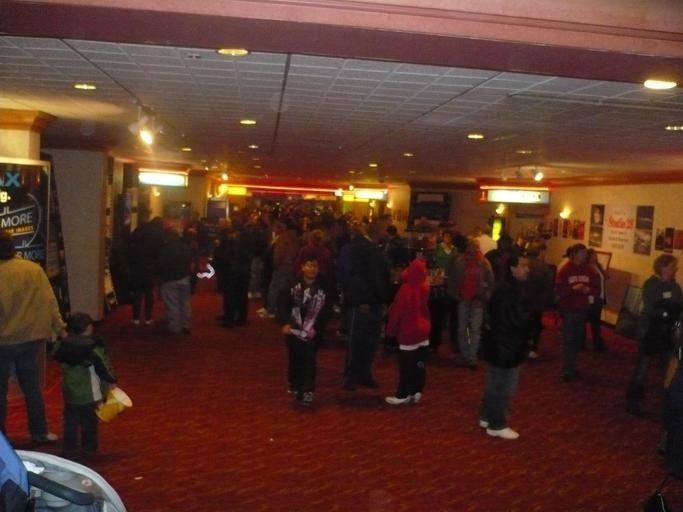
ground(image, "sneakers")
xmin=30 ymin=432 xmax=102 ymax=460
xmin=286 ymin=383 xmax=314 ymax=409
xmin=478 ymin=419 xmax=520 ymax=440
xmin=384 ymin=392 xmax=426 ymax=405
xmin=128 ymin=317 xmax=251 ymax=337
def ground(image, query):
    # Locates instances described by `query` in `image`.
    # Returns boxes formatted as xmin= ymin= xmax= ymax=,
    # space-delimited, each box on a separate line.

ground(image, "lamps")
xmin=501 ymin=162 xmax=544 ymax=182
xmin=127 ymin=104 xmax=163 ymax=146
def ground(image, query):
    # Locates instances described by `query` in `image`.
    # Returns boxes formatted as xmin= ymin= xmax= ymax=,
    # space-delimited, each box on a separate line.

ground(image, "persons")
xmin=416 ymin=222 xmax=681 ymax=478
xmin=126 ymin=203 xmax=433 ymax=407
xmin=49 ymin=313 xmax=117 ymax=460
xmin=0 ymin=230 xmax=68 ymax=443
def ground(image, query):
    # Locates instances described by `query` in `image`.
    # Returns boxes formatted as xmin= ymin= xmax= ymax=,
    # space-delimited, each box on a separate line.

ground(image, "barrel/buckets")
xmin=94 ymin=386 xmax=132 ymax=423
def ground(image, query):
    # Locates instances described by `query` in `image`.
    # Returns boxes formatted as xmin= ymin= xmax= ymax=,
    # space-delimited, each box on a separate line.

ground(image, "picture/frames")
xmin=596 ymin=250 xmax=613 ymax=274
